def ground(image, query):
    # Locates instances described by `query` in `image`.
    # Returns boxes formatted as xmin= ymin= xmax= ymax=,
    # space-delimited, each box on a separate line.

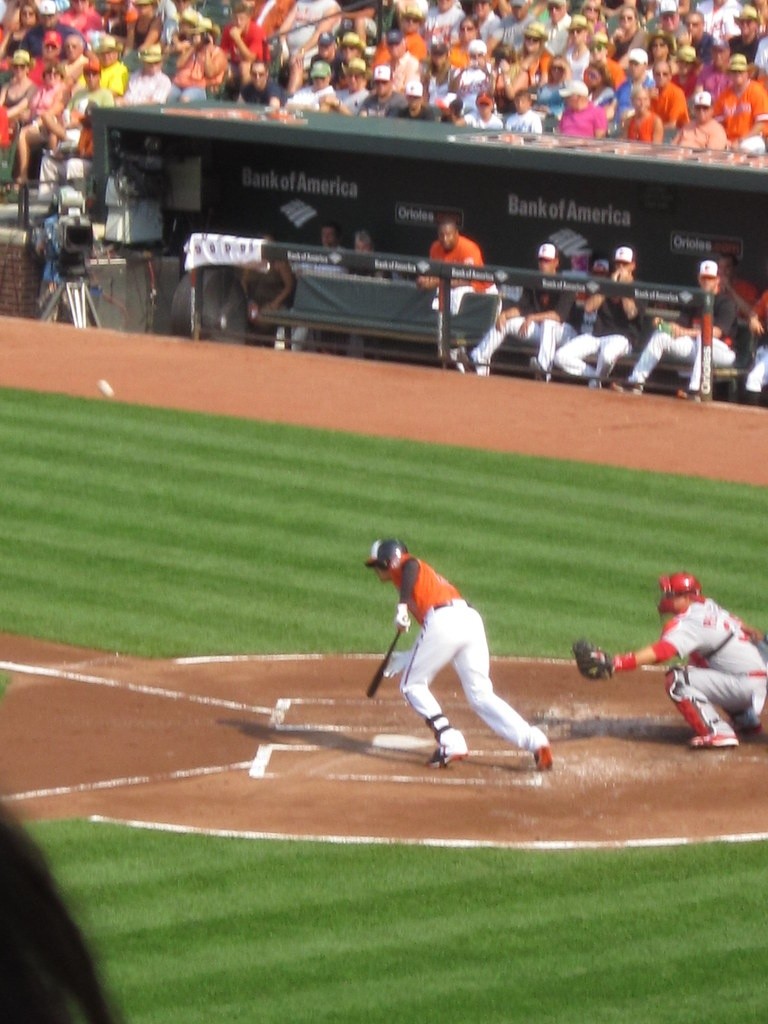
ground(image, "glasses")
xmin=568 ymin=28 xmax=584 ymax=34
xmin=652 ymin=42 xmax=668 ymax=47
xmin=526 ymin=36 xmax=538 ymax=44
xmin=13 ymin=64 xmax=29 ymax=69
xmin=589 ymin=45 xmax=605 ymax=52
xmin=251 ymin=70 xmax=268 ymax=78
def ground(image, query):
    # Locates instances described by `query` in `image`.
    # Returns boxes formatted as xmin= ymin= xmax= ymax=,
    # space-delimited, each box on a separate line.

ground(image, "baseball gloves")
xmin=571 ymin=639 xmax=615 ymax=680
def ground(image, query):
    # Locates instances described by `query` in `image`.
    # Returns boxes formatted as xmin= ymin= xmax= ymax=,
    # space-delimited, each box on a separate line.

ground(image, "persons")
xmin=572 ymin=571 xmax=768 ymax=750
xmin=412 ymin=218 xmax=502 ymax=325
xmin=612 ymin=252 xmax=768 ymax=407
xmin=455 ymin=244 xmax=578 ymax=382
xmin=0 ymin=0 xmax=231 ymax=192
xmin=553 ymin=246 xmax=648 ymax=391
xmin=35 ymin=187 xmax=122 ymax=322
xmin=219 ymin=0 xmax=768 ymax=155
xmin=241 ymin=223 xmax=427 ymax=366
xmin=365 ymin=538 xmax=553 ymax=773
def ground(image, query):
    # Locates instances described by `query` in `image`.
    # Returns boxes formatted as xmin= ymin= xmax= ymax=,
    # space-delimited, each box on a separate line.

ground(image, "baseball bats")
xmin=367 ymin=616 xmax=409 ymax=697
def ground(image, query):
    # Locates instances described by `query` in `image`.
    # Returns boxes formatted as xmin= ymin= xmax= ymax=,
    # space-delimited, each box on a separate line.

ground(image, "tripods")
xmin=40 ymin=262 xmax=102 ymax=329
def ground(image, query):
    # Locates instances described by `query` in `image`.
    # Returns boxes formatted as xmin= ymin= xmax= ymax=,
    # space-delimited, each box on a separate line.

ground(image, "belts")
xmin=434 ymin=601 xmax=469 ymax=609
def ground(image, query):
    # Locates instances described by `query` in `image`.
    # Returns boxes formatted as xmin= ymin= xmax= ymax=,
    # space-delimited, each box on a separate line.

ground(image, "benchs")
xmin=258 ymin=272 xmax=752 ymax=400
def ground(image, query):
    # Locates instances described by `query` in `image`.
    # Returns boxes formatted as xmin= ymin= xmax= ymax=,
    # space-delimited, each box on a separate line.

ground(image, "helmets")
xmin=669 ymin=571 xmax=705 ymax=604
xmin=365 ymin=537 xmax=406 ymax=571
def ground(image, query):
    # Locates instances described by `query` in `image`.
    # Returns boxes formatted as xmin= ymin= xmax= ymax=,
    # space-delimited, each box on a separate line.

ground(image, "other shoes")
xmin=529 ymin=356 xmax=551 ymax=382
xmin=457 ymin=345 xmax=475 ymax=373
xmin=611 ymin=381 xmax=643 ymax=395
xmin=677 ymin=389 xmax=701 ymax=401
xmin=7 ymin=178 xmax=27 ymax=197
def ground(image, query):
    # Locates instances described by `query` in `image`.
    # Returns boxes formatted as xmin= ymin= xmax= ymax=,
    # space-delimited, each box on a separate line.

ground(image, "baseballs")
xmin=96 ymin=379 xmax=114 ymax=396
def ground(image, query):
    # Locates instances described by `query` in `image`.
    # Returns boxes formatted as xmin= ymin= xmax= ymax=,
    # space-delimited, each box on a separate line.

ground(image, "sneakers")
xmin=428 ymin=745 xmax=466 ymax=770
xmin=533 ymin=741 xmax=553 ymax=771
xmin=690 ymin=732 xmax=739 ymax=748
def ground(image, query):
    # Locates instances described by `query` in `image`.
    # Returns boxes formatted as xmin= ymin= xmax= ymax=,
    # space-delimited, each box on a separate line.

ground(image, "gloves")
xmin=394 ymin=604 xmax=411 ymax=630
xmin=383 ymin=650 xmax=411 ymax=678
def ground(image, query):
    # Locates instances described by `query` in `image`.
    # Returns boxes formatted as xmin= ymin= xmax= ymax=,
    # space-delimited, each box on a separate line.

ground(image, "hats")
xmin=537 ymin=243 xmax=558 ymax=261
xmin=696 ymin=260 xmax=718 ymax=278
xmin=312 ymin=5 xmax=761 ymax=109
xmin=12 ymin=0 xmax=221 ymax=75
xmin=612 ymin=245 xmax=635 ymax=264
